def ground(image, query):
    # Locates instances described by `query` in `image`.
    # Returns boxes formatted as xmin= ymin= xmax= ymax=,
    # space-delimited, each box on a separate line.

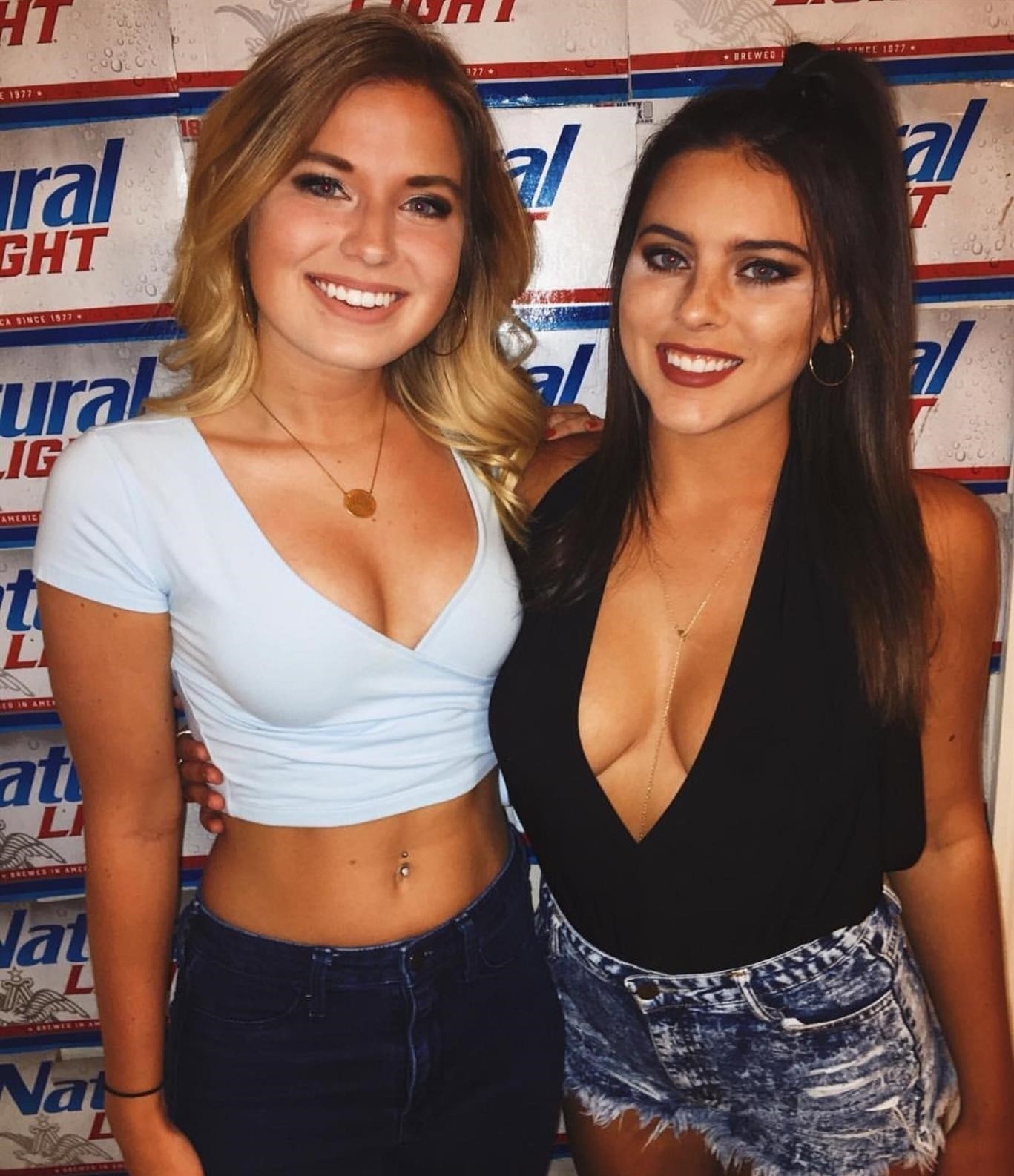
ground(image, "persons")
xmin=31 ymin=6 xmax=608 ymax=1176
xmin=174 ymin=44 xmax=1014 ymax=1176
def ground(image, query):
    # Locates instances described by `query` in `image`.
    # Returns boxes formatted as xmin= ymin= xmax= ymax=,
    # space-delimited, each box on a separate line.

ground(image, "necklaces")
xmin=637 ymin=497 xmax=771 ymax=842
xmin=248 ymin=377 xmax=392 ymax=519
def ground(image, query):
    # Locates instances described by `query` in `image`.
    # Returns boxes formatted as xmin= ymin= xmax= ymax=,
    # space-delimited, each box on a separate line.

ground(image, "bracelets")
xmin=101 ymin=1076 xmax=163 ymax=1098
